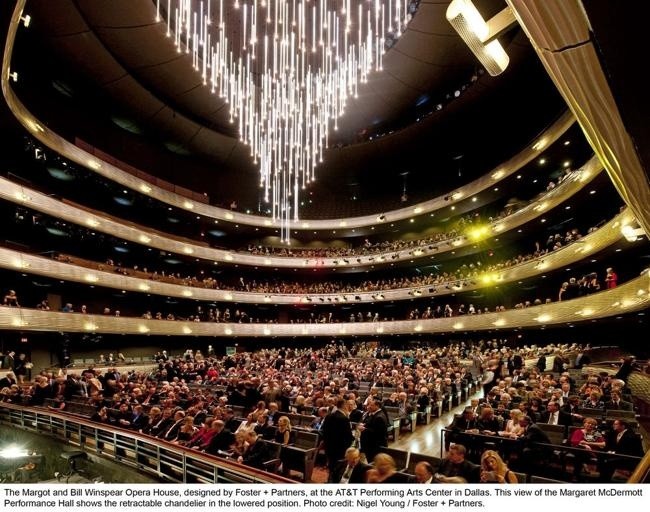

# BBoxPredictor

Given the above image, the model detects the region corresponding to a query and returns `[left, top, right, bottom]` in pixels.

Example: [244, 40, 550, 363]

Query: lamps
[9, 71, 18, 82]
[19, 15, 31, 28]
[150, 0, 414, 246]
[444, 0, 515, 79]
[622, 223, 645, 243]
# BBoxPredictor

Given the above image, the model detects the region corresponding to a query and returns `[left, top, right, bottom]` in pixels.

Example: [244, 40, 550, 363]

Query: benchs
[45, 381, 650, 483]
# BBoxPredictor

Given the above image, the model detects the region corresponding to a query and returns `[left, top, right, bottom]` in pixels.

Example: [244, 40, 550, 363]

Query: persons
[1, 166, 645, 482]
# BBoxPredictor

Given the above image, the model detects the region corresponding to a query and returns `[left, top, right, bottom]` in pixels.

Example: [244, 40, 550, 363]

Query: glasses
[622, 223, 645, 243]
[150, 0, 414, 246]
[444, 0, 515, 79]
[19, 15, 31, 28]
[9, 71, 18, 82]
